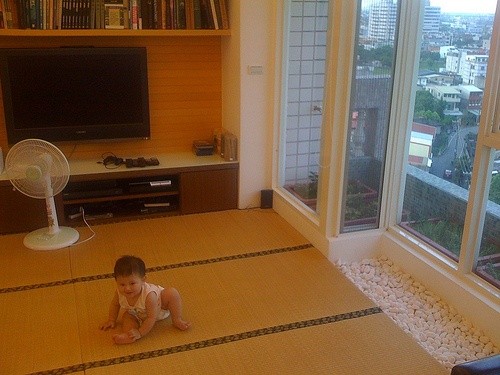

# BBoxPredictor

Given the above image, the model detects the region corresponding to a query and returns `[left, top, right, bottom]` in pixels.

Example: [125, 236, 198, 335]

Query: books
[141, 0, 202, 29]
[91, 0, 142, 30]
[200, 0, 229, 30]
[0, 0, 90, 29]
[213, 128, 237, 160]
[192, 139, 214, 156]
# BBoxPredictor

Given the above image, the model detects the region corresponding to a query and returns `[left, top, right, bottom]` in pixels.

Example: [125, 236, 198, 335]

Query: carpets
[0, 208, 452, 375]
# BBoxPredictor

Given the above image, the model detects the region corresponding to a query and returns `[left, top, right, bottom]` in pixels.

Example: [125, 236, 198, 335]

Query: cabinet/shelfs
[0, 152, 239, 235]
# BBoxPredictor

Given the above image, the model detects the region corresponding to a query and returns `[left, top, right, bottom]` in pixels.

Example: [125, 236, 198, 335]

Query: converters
[125, 160, 147, 168]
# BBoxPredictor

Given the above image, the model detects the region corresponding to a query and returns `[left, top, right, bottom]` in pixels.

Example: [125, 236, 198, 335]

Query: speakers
[260, 188, 274, 209]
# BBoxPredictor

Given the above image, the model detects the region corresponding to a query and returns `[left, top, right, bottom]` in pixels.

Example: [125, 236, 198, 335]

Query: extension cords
[68, 210, 85, 219]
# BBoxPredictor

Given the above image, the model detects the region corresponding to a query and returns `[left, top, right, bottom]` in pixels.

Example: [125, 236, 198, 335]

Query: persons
[98, 255, 190, 345]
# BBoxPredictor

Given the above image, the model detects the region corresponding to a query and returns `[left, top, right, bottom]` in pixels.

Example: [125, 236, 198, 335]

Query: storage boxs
[192, 140, 215, 156]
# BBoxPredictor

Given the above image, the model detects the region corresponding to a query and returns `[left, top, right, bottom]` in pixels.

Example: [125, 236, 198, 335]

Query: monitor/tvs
[0, 45, 152, 148]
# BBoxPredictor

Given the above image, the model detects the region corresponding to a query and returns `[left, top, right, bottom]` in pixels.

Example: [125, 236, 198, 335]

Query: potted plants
[283, 171, 500, 289]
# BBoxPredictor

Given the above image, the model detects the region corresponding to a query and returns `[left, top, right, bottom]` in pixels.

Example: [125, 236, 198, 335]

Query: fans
[5, 139, 80, 251]
